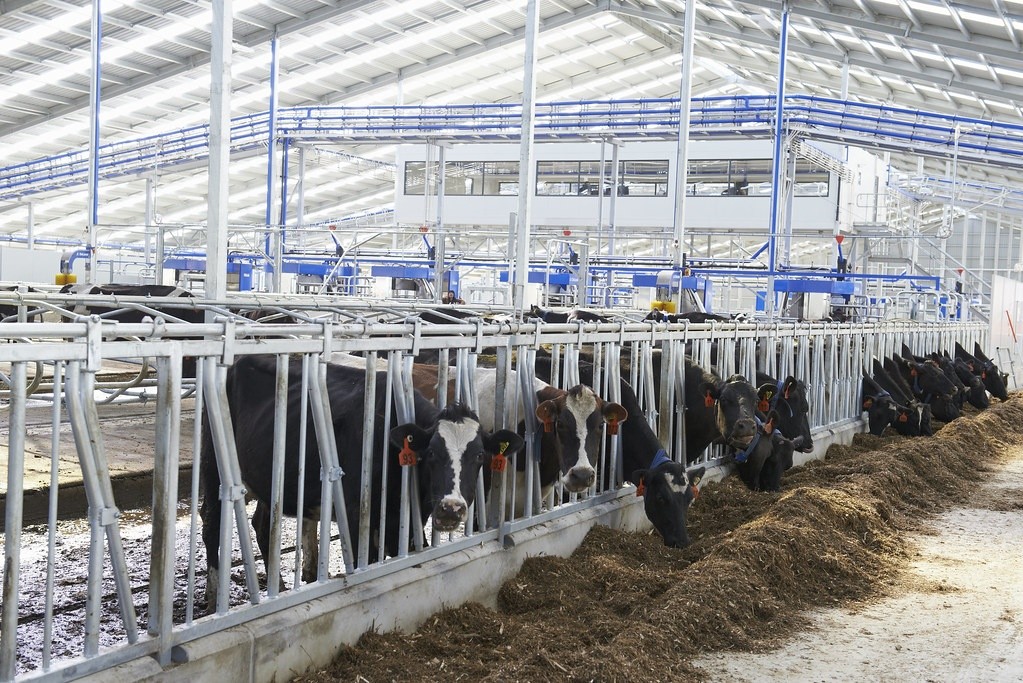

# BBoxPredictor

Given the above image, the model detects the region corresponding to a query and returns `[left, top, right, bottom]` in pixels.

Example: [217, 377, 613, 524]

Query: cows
[0, 282, 1011, 616]
